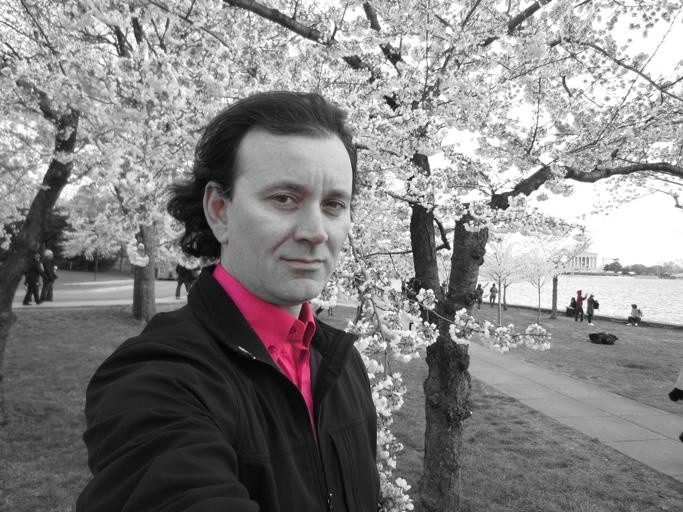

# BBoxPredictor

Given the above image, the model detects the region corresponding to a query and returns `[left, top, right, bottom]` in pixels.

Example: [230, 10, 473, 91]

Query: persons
[22, 251, 49, 305]
[490, 283, 498, 307]
[189, 264, 202, 281]
[475, 283, 483, 310]
[626, 303, 642, 326]
[574, 290, 587, 322]
[175, 264, 193, 299]
[569, 297, 576, 310]
[667, 367, 683, 444]
[75, 89, 381, 512]
[40, 248, 58, 301]
[587, 292, 595, 326]
